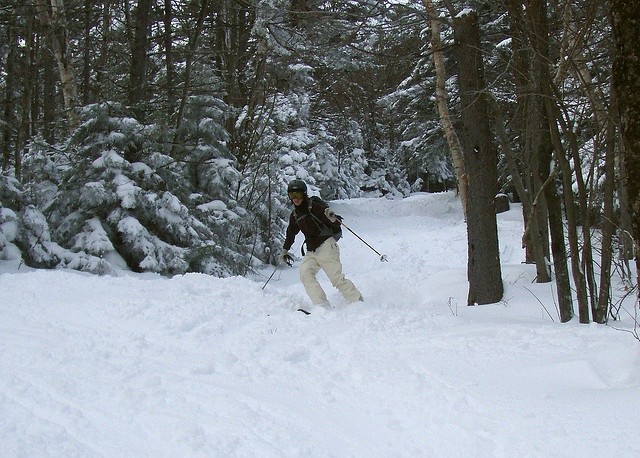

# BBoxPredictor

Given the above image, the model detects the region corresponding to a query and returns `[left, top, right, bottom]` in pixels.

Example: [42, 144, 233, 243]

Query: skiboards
[297, 309, 311, 315]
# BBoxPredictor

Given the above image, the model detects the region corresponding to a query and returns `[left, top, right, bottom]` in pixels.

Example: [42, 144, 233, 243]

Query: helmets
[288, 181, 307, 197]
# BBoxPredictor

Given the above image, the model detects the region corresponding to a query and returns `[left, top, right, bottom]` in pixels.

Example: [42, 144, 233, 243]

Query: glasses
[288, 191, 304, 200]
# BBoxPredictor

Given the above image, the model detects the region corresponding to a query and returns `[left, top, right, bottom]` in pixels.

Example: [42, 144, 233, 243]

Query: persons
[280, 180, 364, 309]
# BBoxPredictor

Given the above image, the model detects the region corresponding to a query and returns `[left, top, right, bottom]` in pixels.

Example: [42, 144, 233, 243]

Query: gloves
[277, 249, 290, 265]
[324, 208, 336, 223]
